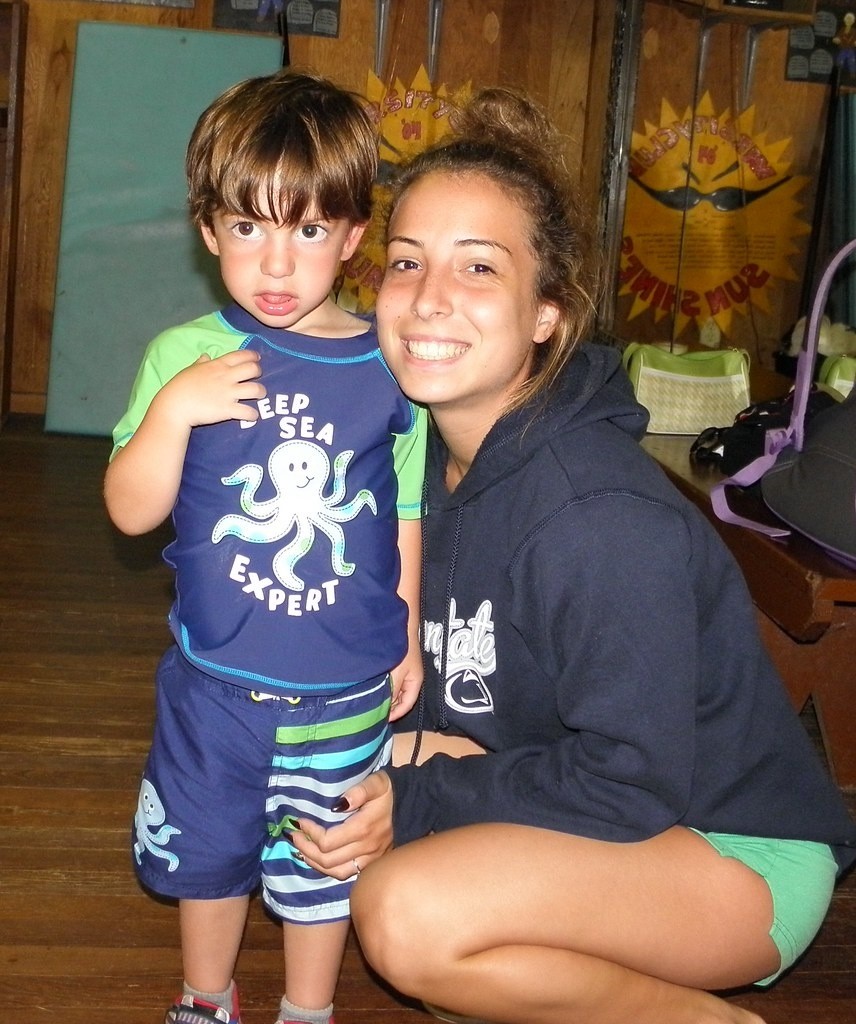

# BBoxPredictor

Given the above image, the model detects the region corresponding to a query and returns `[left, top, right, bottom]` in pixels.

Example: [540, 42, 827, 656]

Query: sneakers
[163, 984, 241, 1024]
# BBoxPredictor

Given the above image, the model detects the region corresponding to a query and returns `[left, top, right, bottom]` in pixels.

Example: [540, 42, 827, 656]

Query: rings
[351, 858, 361, 875]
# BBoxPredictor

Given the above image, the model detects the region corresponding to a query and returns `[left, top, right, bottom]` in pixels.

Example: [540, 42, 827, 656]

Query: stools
[637, 428, 856, 796]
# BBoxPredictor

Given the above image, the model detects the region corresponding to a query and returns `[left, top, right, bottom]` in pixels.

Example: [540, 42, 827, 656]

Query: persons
[103, 71, 429, 1024]
[282, 86, 855, 1024]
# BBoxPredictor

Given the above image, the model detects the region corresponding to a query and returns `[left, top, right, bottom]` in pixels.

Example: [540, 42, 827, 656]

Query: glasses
[690, 427, 733, 453]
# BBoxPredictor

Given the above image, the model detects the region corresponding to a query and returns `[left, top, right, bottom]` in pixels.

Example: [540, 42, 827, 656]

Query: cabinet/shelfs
[0, 0, 848, 419]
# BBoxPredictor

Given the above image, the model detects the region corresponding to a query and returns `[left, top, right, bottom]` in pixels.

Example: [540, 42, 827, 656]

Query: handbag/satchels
[819, 353, 856, 398]
[622, 340, 751, 434]
